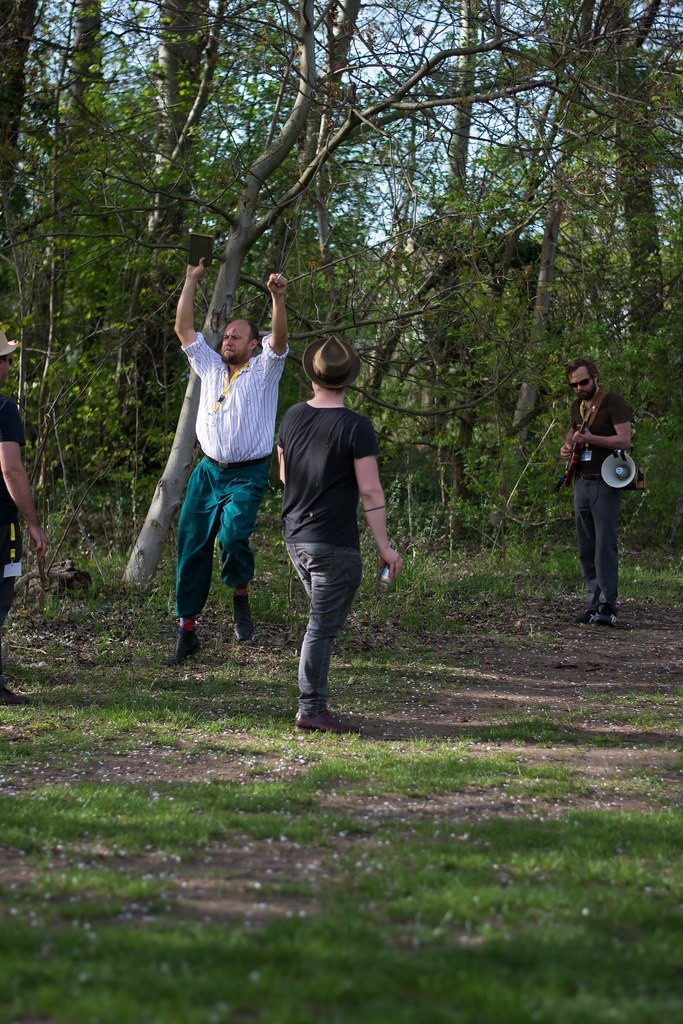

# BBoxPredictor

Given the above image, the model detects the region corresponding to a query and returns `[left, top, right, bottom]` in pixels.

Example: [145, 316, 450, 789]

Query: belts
[575, 473, 602, 480]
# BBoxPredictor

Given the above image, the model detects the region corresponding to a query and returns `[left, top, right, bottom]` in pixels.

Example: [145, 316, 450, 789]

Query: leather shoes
[295, 709, 361, 737]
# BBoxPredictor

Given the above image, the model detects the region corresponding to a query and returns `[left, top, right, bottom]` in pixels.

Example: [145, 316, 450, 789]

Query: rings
[36, 542, 42, 547]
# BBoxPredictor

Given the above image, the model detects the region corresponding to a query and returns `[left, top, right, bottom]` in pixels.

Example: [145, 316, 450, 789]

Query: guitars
[564, 406, 596, 488]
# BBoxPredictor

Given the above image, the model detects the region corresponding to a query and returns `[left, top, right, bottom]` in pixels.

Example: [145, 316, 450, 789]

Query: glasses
[568, 376, 593, 388]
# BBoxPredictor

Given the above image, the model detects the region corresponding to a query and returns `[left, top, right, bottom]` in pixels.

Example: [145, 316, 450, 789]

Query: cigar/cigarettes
[276, 272, 284, 279]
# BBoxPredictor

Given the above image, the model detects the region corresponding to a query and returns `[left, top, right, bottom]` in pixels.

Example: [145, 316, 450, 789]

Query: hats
[303, 336, 360, 389]
[0, 330, 18, 356]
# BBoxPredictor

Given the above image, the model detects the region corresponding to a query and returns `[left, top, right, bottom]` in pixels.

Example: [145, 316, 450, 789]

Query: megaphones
[601, 452, 639, 489]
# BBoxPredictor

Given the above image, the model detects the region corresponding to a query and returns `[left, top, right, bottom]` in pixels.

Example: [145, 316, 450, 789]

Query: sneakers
[166, 633, 199, 665]
[233, 593, 253, 641]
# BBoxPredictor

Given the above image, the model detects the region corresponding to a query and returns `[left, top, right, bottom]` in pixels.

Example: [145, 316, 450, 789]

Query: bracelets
[365, 505, 385, 512]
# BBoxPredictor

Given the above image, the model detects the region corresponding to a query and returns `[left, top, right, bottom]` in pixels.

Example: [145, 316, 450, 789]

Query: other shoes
[574, 610, 600, 624]
[594, 614, 615, 624]
[0, 685, 29, 704]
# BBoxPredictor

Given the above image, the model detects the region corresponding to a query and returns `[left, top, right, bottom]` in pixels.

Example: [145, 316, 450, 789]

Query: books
[188, 232, 212, 266]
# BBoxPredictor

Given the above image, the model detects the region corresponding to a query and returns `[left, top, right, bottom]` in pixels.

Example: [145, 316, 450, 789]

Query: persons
[275, 337, 404, 736]
[559, 357, 635, 628]
[0, 326, 48, 706]
[174, 256, 289, 661]
